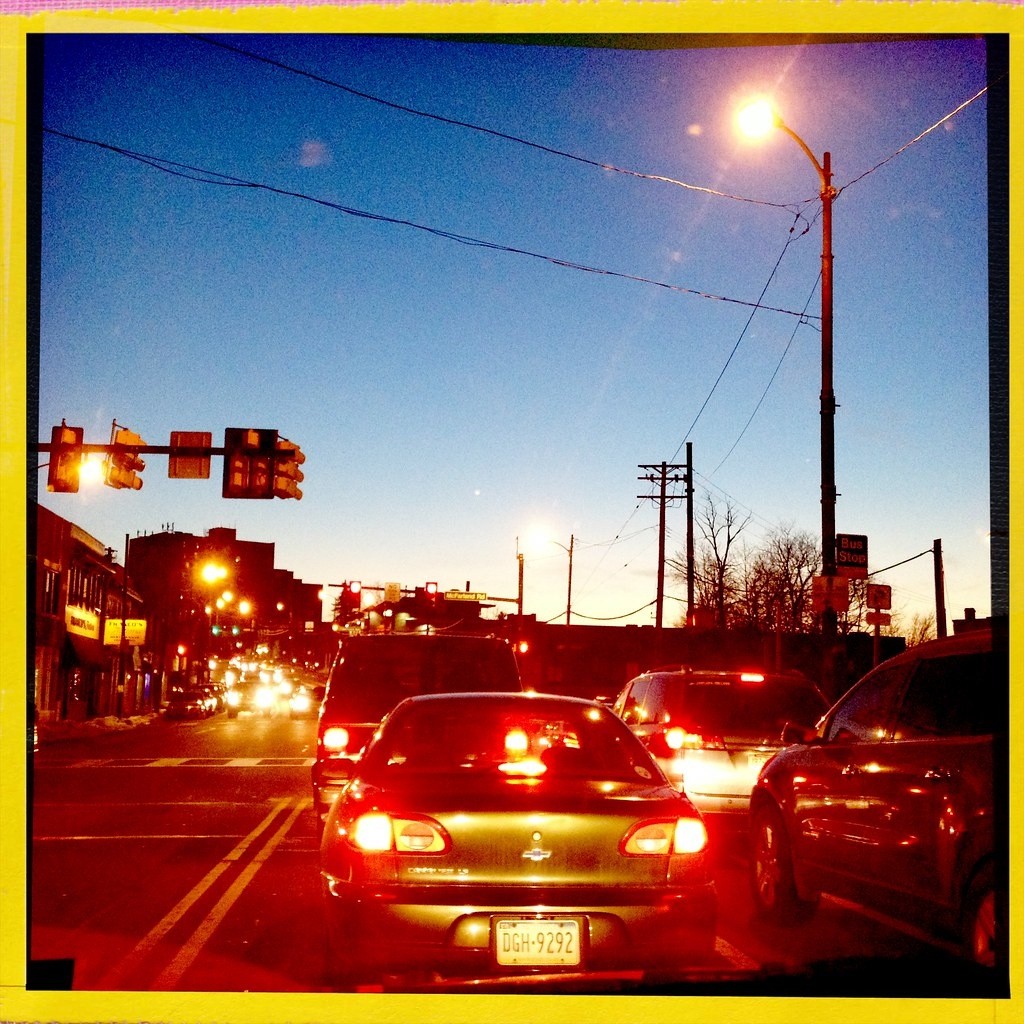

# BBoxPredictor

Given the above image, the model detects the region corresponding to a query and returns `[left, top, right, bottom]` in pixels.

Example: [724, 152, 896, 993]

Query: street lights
[728, 92, 837, 706]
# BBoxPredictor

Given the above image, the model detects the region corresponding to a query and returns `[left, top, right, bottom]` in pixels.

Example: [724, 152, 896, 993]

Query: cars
[167, 646, 624, 722]
[317, 693, 714, 993]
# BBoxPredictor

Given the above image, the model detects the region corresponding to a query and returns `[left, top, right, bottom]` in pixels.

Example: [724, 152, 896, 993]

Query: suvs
[747, 624, 1002, 984]
[311, 631, 539, 844]
[605, 664, 840, 820]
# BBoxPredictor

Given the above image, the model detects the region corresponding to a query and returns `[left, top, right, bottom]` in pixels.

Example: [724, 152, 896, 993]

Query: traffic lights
[111, 429, 148, 490]
[274, 438, 306, 501]
